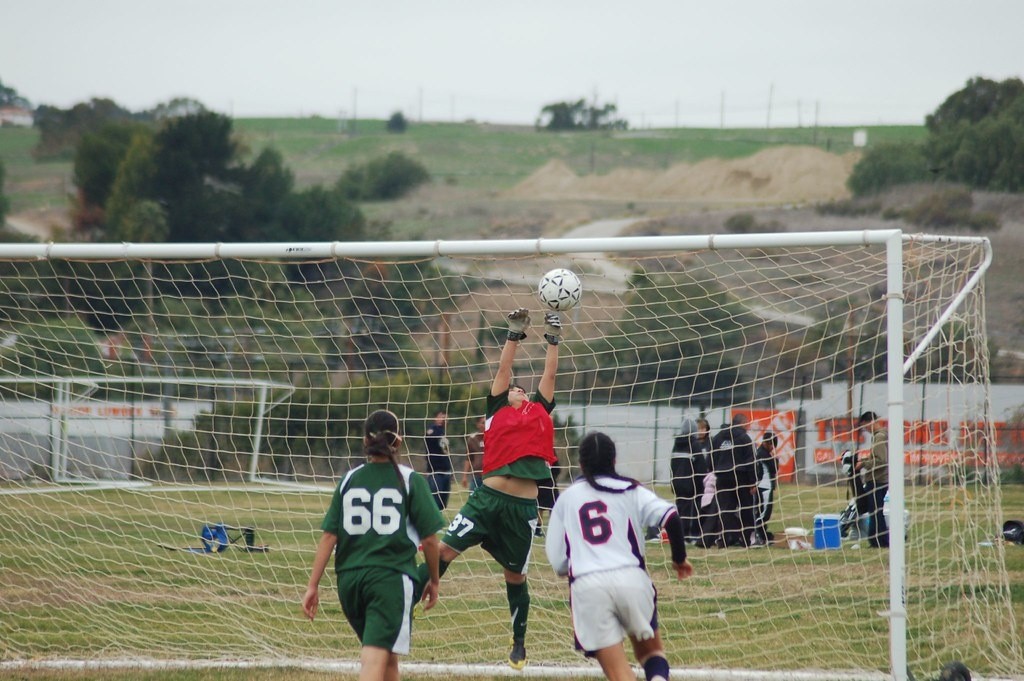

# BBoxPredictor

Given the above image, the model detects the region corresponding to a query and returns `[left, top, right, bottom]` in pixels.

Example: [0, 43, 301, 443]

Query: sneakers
[507, 636, 526, 669]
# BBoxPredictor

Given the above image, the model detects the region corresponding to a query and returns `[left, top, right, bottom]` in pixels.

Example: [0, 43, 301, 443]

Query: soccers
[539, 267, 583, 311]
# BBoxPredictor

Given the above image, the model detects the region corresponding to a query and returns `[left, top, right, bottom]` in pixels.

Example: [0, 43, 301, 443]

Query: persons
[546, 430, 694, 681]
[302, 409, 447, 681]
[422, 408, 561, 540]
[840, 412, 889, 550]
[670, 413, 779, 549]
[415, 307, 561, 665]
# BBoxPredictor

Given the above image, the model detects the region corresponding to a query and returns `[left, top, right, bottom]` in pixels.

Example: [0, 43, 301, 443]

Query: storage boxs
[812, 513, 842, 549]
[772, 530, 813, 548]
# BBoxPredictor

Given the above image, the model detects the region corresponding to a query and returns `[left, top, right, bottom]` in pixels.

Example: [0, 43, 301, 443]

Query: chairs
[183, 528, 248, 555]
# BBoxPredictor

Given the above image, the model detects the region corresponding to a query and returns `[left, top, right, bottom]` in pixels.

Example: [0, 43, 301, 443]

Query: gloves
[543, 312, 562, 346]
[506, 310, 533, 342]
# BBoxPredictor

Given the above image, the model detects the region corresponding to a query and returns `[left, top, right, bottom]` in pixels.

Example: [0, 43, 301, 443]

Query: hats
[682, 417, 698, 436]
